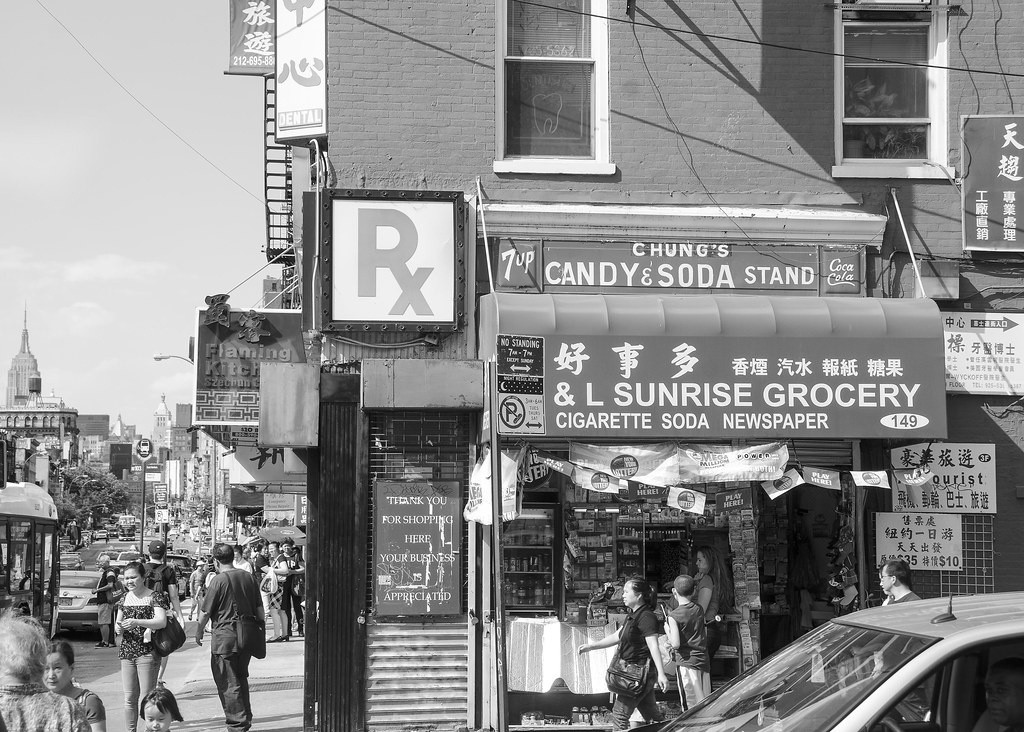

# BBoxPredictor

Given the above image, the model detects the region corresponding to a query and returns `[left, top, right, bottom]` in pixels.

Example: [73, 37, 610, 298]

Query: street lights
[82, 480, 100, 490]
[152, 354, 217, 551]
[68, 474, 89, 498]
[20, 451, 48, 483]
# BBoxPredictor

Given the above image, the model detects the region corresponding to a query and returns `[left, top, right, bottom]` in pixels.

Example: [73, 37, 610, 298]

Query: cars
[42, 570, 103, 636]
[63, 514, 237, 602]
[59, 551, 86, 571]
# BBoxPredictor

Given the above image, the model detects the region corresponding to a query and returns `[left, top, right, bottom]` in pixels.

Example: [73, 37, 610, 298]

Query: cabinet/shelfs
[502, 544, 554, 612]
[655, 614, 739, 691]
[568, 511, 727, 593]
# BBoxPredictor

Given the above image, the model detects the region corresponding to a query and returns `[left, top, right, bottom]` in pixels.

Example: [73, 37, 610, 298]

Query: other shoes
[299, 631, 304, 637]
[94, 642, 109, 649]
[188, 614, 192, 620]
[288, 630, 292, 636]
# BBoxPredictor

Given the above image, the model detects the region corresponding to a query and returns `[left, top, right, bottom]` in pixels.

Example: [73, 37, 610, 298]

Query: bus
[0, 482, 63, 644]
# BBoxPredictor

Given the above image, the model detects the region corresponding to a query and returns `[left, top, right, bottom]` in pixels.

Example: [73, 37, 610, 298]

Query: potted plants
[845, 74, 927, 158]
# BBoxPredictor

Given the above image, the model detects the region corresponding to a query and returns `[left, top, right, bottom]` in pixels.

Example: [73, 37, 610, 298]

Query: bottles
[503, 524, 553, 606]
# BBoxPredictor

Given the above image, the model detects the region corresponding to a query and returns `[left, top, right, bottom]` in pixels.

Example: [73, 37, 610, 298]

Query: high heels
[282, 635, 289, 641]
[266, 636, 282, 643]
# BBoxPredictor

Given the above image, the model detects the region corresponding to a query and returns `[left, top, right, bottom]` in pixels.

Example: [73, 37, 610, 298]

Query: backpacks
[142, 563, 168, 591]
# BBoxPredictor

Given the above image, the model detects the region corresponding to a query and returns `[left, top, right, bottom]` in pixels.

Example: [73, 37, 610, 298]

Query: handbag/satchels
[605, 650, 651, 700]
[236, 614, 266, 659]
[150, 591, 186, 657]
[292, 574, 305, 596]
[105, 569, 125, 605]
[259, 569, 278, 595]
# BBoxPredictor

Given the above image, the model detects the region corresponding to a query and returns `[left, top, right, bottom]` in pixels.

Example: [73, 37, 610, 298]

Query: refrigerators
[491, 501, 562, 619]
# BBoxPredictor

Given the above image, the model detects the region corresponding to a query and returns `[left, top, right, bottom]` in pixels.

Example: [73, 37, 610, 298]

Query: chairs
[972, 640, 1024, 732]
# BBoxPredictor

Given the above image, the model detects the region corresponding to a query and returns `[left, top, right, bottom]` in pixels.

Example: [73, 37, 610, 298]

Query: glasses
[881, 575, 894, 579]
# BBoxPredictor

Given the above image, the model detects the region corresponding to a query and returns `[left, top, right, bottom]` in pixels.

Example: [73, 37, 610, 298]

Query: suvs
[657, 590, 1024, 732]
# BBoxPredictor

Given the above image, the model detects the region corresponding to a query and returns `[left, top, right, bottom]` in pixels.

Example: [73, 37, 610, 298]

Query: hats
[280, 537, 294, 547]
[196, 560, 206, 566]
[826, 525, 859, 615]
[149, 540, 166, 555]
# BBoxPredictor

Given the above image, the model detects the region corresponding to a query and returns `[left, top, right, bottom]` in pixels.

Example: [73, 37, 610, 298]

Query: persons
[189, 537, 306, 646]
[664, 574, 711, 710]
[196, 543, 265, 732]
[140, 681, 183, 732]
[41, 641, 106, 732]
[578, 577, 670, 732]
[851, 558, 938, 723]
[986, 656, 1024, 732]
[105, 532, 110, 544]
[171, 515, 211, 545]
[141, 540, 185, 689]
[115, 562, 167, 732]
[0, 608, 92, 732]
[663, 545, 732, 694]
[92, 554, 115, 649]
